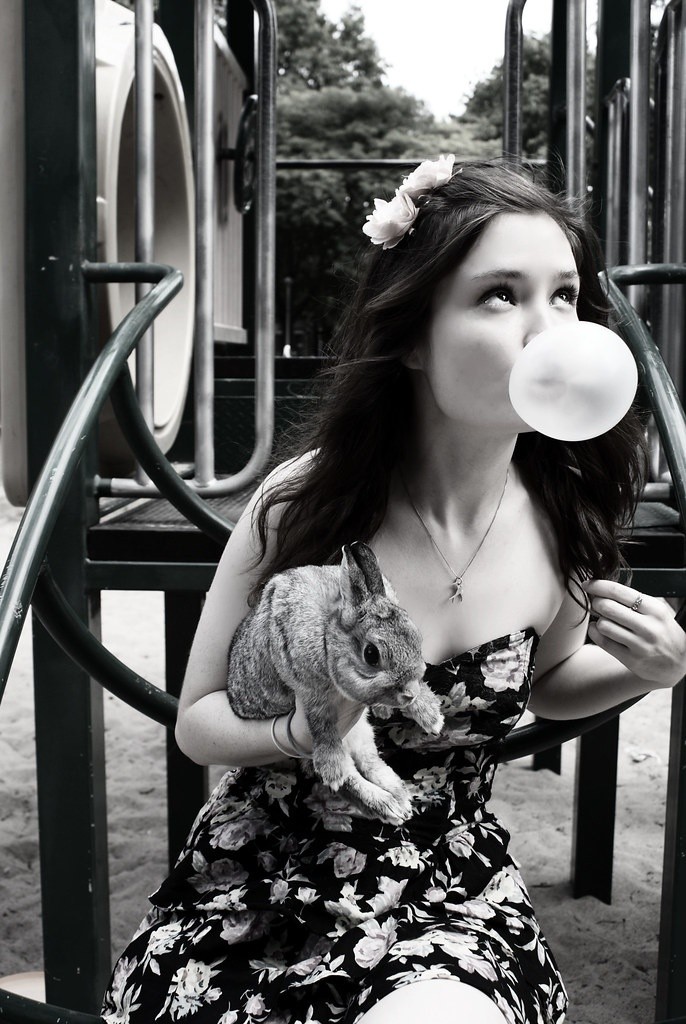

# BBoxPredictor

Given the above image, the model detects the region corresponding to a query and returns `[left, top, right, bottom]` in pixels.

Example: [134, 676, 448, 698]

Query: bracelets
[285, 708, 313, 759]
[270, 716, 304, 758]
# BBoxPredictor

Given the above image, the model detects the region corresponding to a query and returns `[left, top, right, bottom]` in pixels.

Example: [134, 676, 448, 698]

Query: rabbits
[226, 539, 445, 826]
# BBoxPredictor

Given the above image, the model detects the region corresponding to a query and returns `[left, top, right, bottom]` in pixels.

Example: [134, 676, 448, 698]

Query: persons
[99, 164, 686, 1024]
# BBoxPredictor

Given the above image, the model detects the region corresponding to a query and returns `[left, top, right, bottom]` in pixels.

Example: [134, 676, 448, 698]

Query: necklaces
[403, 467, 509, 603]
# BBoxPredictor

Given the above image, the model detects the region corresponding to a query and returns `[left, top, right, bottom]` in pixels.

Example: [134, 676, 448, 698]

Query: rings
[632, 593, 645, 610]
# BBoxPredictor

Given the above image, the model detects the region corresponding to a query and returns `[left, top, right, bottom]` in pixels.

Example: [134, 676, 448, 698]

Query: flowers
[362, 192, 421, 251]
[398, 154, 462, 198]
